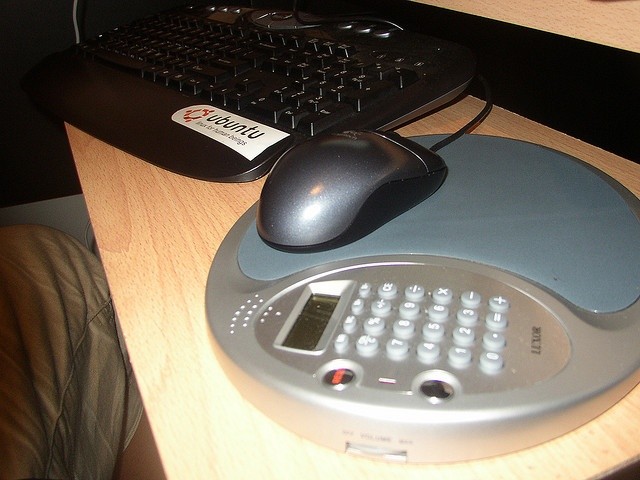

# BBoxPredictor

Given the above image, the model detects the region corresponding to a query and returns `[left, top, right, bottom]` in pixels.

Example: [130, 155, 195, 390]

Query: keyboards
[25, 11, 474, 183]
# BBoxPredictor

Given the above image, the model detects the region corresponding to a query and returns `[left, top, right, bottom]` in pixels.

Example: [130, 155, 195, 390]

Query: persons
[2, 222, 168, 480]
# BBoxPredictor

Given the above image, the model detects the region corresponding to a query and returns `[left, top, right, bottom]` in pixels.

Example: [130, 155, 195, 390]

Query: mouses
[256, 130, 446, 254]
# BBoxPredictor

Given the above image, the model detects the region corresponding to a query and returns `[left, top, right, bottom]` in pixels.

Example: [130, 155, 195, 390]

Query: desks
[64, 92, 640, 480]
